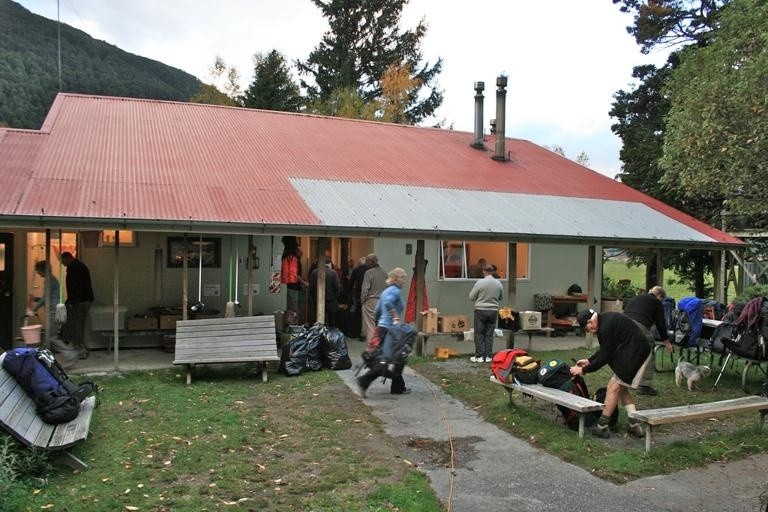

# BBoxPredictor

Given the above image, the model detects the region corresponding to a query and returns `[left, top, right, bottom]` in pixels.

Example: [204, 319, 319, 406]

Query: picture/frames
[167, 236, 221, 268]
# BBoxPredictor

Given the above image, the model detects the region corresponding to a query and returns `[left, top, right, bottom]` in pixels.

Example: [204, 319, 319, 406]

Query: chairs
[655, 297, 768, 392]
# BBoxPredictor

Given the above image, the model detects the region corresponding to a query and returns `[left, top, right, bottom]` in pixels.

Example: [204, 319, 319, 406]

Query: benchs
[0, 359, 97, 471]
[490, 376, 607, 439]
[631, 395, 768, 454]
[418, 329, 520, 357]
[103, 330, 175, 353]
[172, 315, 280, 385]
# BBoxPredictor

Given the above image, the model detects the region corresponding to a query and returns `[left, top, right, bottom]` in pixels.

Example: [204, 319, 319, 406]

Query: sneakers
[391, 387, 412, 395]
[589, 424, 609, 439]
[485, 356, 492, 363]
[624, 422, 645, 439]
[636, 385, 657, 396]
[354, 378, 368, 399]
[470, 356, 485, 363]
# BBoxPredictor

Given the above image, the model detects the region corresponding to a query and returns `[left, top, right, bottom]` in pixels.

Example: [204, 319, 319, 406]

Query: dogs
[674, 356, 714, 391]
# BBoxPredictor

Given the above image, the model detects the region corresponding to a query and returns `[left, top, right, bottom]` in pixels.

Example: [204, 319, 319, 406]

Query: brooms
[223, 234, 236, 319]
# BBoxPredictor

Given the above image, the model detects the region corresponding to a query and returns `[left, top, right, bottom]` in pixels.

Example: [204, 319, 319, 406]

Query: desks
[535, 295, 596, 337]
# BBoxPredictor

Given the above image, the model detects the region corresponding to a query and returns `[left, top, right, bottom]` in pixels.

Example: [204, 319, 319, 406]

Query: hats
[576, 309, 595, 328]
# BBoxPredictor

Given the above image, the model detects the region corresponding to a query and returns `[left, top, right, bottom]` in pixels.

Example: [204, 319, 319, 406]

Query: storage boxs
[419, 307, 440, 333]
[89, 306, 190, 331]
[519, 311, 542, 328]
[438, 314, 468, 332]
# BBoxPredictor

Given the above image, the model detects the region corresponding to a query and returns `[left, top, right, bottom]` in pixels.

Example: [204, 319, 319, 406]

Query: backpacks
[594, 388, 620, 433]
[352, 322, 418, 384]
[536, 357, 594, 434]
[646, 295, 768, 366]
[1, 345, 96, 425]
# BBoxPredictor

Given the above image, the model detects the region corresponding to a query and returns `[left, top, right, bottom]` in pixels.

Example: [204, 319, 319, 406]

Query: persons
[62, 252, 94, 359]
[468, 265, 504, 363]
[353, 267, 411, 398]
[287, 247, 389, 352]
[569, 309, 653, 438]
[457, 259, 501, 279]
[33, 261, 60, 340]
[623, 286, 673, 396]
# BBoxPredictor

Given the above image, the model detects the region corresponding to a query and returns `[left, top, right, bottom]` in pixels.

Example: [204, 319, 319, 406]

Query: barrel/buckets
[18, 312, 44, 345]
